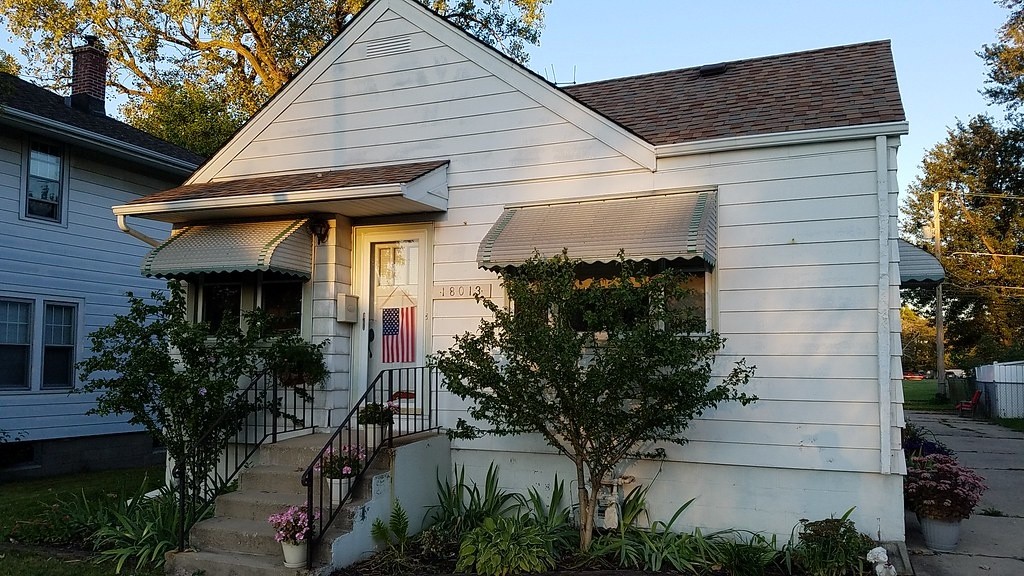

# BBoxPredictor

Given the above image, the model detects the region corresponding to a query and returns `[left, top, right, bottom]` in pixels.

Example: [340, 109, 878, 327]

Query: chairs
[956, 390, 982, 420]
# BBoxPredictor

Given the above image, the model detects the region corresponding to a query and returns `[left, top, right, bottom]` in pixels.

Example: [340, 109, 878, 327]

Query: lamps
[307, 217, 334, 246]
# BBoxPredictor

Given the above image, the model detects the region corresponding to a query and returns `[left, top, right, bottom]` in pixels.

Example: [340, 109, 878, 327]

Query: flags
[382, 305, 416, 363]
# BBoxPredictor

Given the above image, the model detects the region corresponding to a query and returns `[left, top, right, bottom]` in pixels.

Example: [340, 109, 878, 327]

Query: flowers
[903, 452, 991, 523]
[292, 443, 369, 479]
[359, 401, 399, 426]
[386, 390, 415, 402]
[269, 502, 325, 548]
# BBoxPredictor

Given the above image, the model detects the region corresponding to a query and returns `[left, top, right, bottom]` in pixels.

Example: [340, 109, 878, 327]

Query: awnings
[476, 185, 717, 272]
[900, 237, 946, 289]
[141, 219, 313, 280]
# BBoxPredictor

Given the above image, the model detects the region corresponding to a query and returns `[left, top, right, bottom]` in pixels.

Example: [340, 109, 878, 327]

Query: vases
[920, 515, 962, 551]
[393, 398, 414, 414]
[326, 475, 357, 505]
[281, 538, 308, 569]
[363, 423, 387, 448]
